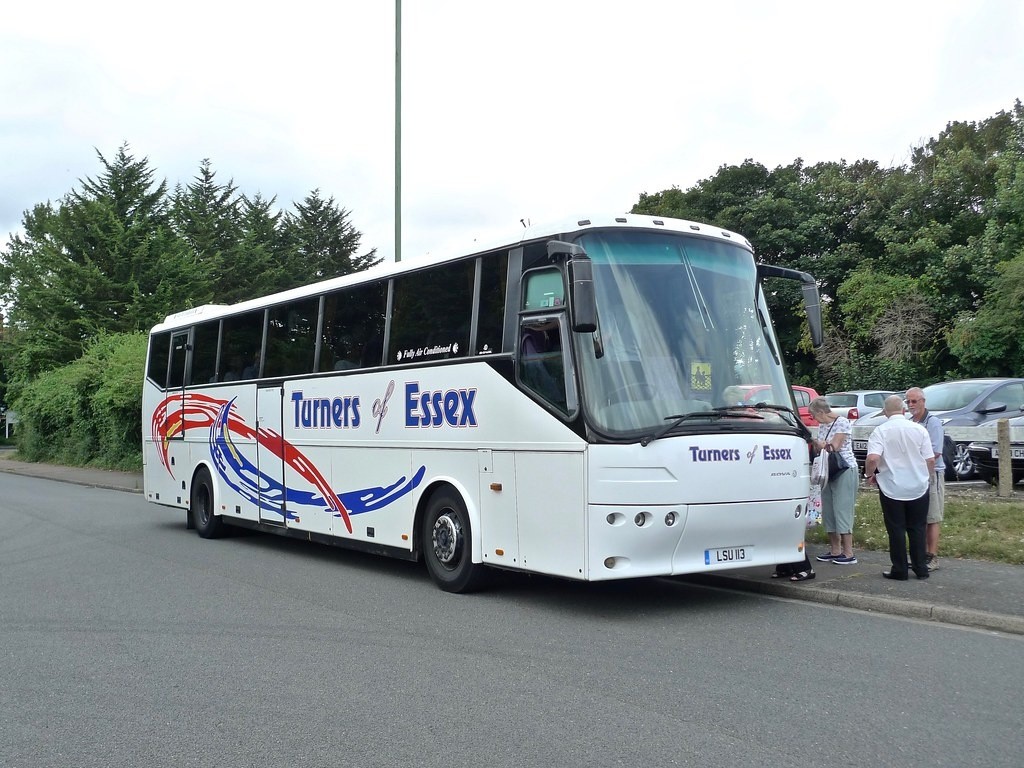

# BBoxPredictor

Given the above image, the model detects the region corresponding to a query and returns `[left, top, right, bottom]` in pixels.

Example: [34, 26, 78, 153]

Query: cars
[965, 407, 1024, 481]
[726, 385, 823, 460]
[826, 389, 908, 429]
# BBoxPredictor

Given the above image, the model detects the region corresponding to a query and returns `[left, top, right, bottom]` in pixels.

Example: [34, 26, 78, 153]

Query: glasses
[903, 398, 924, 404]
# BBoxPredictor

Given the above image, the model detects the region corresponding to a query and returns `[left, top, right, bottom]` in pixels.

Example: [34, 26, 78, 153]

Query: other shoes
[883, 571, 897, 579]
[916, 574, 929, 580]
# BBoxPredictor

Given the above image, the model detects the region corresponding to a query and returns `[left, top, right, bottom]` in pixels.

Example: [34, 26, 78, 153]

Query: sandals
[771, 570, 792, 578]
[790, 569, 815, 581]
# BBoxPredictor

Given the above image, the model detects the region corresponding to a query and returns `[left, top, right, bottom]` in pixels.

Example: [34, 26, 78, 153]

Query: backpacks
[926, 416, 957, 478]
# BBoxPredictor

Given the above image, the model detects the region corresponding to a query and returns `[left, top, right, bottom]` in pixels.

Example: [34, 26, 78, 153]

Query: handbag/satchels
[811, 442, 849, 491]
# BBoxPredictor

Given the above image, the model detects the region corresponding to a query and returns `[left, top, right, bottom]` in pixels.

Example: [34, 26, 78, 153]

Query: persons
[771, 550, 816, 581]
[904, 387, 946, 572]
[334, 337, 382, 370]
[864, 395, 936, 581]
[807, 396, 857, 564]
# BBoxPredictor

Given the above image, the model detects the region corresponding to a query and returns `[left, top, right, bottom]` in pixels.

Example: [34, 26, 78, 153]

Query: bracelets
[864, 473, 871, 479]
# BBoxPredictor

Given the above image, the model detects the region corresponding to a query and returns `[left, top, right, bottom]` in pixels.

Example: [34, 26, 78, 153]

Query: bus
[141, 214, 825, 596]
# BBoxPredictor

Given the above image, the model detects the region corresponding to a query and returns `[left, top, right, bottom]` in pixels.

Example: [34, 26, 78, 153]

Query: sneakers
[908, 552, 940, 572]
[833, 555, 857, 564]
[817, 552, 843, 561]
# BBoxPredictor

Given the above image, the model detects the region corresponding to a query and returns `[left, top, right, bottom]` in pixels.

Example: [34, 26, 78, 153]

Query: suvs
[849, 378, 1023, 481]
